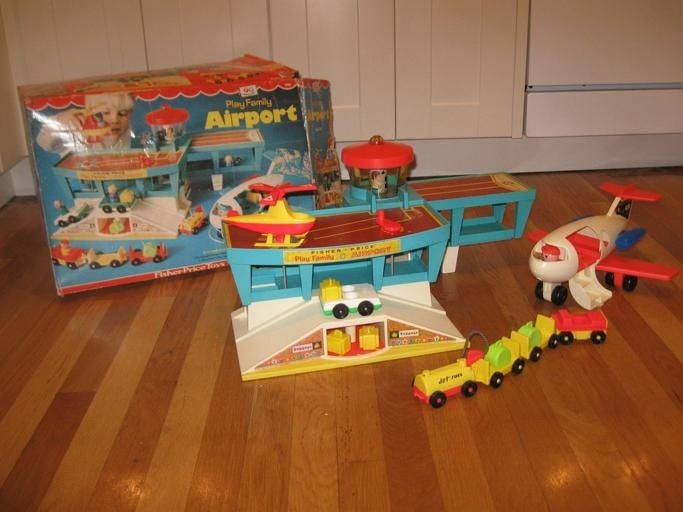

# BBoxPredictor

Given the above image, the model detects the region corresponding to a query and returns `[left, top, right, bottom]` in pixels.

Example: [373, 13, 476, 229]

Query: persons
[35, 82, 136, 158]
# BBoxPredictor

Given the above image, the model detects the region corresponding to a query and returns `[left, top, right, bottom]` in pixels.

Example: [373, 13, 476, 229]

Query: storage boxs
[14, 53, 347, 305]
